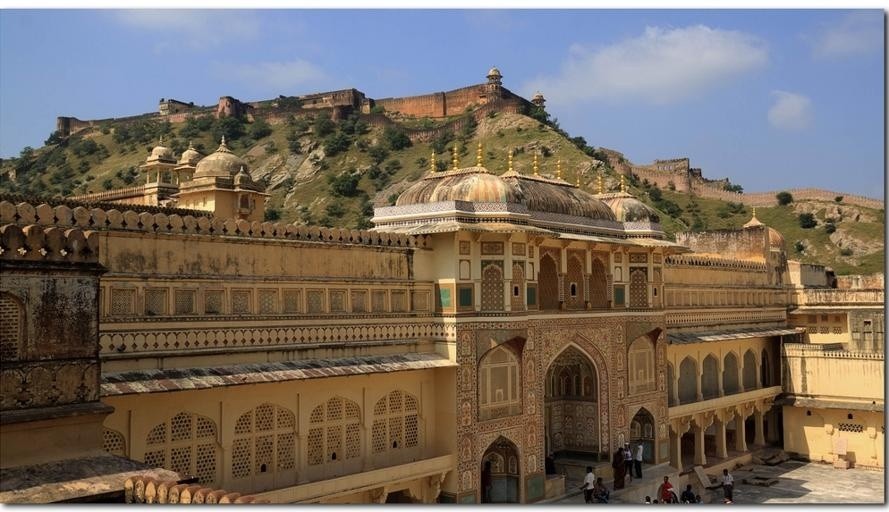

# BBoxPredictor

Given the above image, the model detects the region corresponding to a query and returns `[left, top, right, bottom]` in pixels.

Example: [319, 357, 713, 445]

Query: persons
[612, 447, 625, 489]
[622, 441, 634, 482]
[480, 460, 493, 502]
[578, 465, 596, 503]
[592, 476, 610, 503]
[543, 453, 559, 475]
[720, 468, 734, 503]
[659, 475, 674, 500]
[645, 483, 704, 504]
[633, 438, 644, 479]
